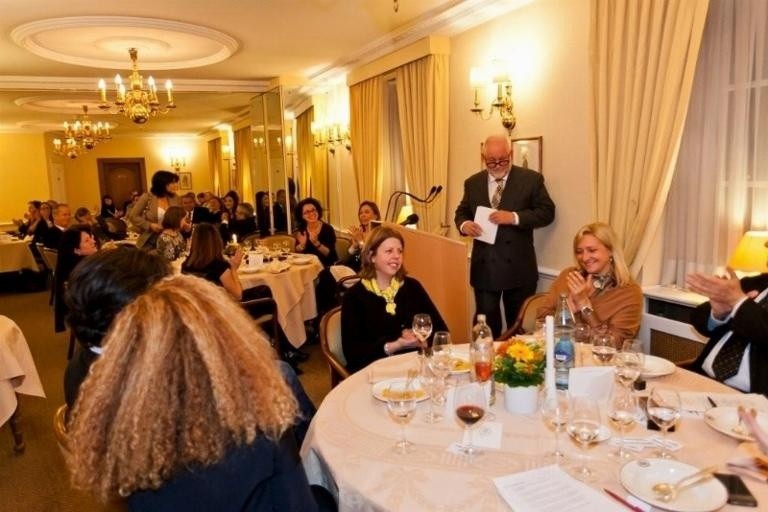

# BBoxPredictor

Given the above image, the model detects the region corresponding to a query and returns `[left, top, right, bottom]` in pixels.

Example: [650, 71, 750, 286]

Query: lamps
[49, 50, 175, 160]
[470, 70, 517, 130]
[310, 122, 341, 157]
[717, 232, 768, 278]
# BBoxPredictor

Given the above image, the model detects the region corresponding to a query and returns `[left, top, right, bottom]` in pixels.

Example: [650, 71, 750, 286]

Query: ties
[711, 287, 767, 382]
[491, 177, 504, 208]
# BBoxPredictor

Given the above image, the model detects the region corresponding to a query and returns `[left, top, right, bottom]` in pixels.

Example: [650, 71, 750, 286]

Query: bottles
[467, 314, 494, 383]
[552, 333, 574, 389]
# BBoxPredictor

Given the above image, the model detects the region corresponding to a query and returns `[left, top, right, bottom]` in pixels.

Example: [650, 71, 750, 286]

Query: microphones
[399, 213, 419, 226]
[384, 185, 436, 221]
[390, 185, 443, 223]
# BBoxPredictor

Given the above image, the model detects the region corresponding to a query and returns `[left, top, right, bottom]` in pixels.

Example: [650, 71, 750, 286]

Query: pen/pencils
[602, 488, 645, 512]
[707, 396, 717, 408]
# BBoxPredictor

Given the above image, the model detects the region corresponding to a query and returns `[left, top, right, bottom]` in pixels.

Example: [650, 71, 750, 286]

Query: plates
[623, 351, 675, 378]
[621, 454, 727, 512]
[427, 350, 471, 375]
[372, 377, 428, 403]
[704, 405, 767, 444]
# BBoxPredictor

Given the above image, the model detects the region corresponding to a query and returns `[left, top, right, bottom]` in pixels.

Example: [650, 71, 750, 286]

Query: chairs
[256, 235, 297, 253]
[36, 243, 59, 307]
[333, 237, 352, 265]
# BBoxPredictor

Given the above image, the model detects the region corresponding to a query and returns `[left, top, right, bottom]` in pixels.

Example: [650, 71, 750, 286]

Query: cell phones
[713, 473, 757, 507]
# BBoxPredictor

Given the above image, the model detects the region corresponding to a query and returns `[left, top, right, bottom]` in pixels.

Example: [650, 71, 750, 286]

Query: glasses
[483, 155, 510, 168]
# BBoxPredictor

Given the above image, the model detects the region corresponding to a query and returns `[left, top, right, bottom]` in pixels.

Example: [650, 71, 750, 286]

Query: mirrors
[249, 84, 330, 238]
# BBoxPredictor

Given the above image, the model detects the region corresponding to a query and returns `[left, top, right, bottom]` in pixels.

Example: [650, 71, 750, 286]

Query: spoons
[650, 462, 716, 496]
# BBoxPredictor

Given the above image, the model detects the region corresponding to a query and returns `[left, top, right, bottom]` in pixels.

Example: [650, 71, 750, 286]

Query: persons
[454, 132, 556, 341]
[63, 244, 318, 453]
[534, 221, 646, 352]
[684, 237, 767, 400]
[338, 224, 453, 372]
[7, 167, 383, 378]
[58, 272, 341, 511]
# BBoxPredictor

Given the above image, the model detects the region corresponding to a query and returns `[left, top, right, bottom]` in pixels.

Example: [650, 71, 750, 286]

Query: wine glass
[452, 377, 487, 458]
[386, 380, 419, 455]
[411, 314, 452, 424]
[542, 321, 682, 484]
[222, 234, 315, 274]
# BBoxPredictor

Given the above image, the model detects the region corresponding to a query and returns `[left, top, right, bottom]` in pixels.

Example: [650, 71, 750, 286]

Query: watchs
[579, 305, 594, 316]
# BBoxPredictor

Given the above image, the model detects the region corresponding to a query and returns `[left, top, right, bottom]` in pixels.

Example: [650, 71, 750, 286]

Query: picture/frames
[179, 171, 192, 190]
[513, 135, 543, 177]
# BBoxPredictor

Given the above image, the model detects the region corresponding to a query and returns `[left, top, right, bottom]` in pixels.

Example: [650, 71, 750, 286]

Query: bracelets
[383, 343, 391, 356]
